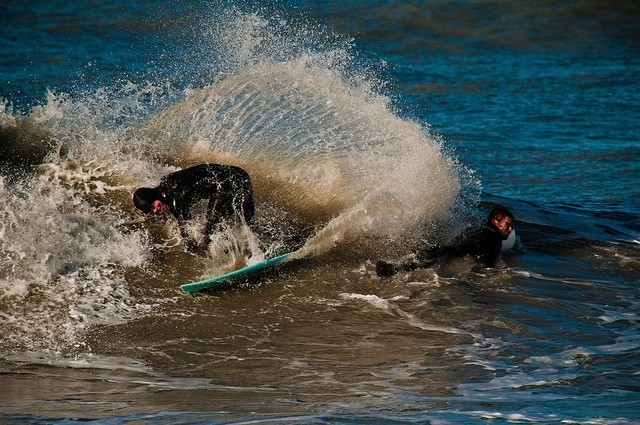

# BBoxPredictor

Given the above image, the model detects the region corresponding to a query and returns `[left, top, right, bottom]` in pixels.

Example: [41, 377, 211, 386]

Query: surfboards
[180, 252, 293, 292]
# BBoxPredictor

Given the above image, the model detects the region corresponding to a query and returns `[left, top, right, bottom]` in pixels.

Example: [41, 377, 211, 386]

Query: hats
[133, 187, 160, 214]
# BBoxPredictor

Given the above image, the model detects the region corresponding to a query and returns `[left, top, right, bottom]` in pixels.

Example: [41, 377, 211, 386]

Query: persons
[134, 164, 255, 250]
[376, 208, 515, 280]
